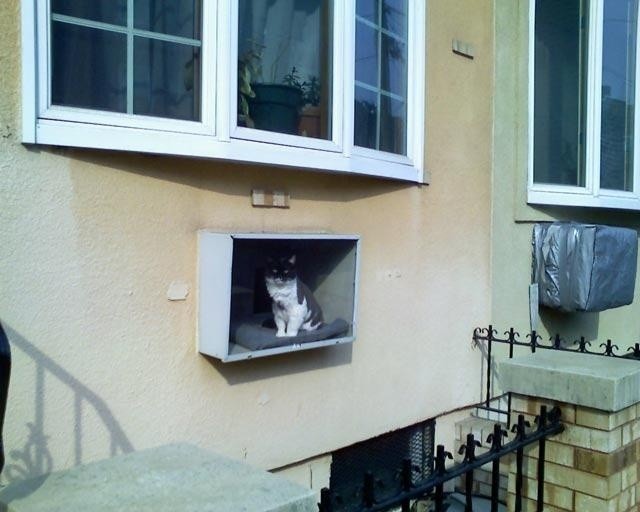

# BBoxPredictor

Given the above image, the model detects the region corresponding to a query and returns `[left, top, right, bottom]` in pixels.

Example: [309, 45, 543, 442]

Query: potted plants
[238, 30, 320, 138]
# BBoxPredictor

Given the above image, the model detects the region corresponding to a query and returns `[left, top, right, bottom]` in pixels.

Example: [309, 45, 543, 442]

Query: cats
[261, 253, 323, 338]
